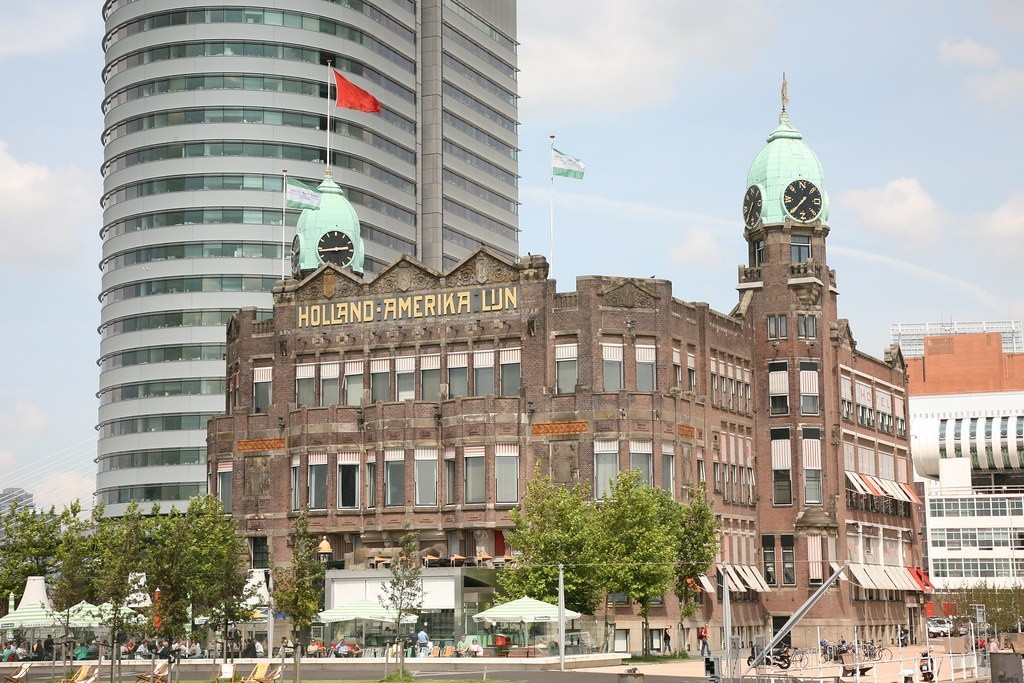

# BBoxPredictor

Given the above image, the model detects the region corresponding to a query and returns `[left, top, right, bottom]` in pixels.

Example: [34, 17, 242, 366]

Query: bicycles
[816, 638, 893, 664]
[780, 641, 808, 668]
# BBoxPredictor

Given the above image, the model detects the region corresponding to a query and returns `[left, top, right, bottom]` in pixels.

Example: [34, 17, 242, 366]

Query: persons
[407, 626, 433, 657]
[352, 638, 363, 657]
[701, 624, 712, 656]
[214, 626, 265, 657]
[663, 628, 672, 654]
[124, 637, 202, 659]
[0, 634, 112, 662]
[334, 639, 348, 658]
[457, 635, 469, 657]
[901, 630, 906, 646]
[277, 637, 304, 658]
[307, 639, 318, 657]
[463, 638, 483, 656]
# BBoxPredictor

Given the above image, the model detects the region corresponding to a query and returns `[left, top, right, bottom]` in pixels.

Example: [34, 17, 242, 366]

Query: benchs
[839, 653, 873, 677]
[473, 654, 492, 657]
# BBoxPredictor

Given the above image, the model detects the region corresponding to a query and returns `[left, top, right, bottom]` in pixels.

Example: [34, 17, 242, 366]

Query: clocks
[743, 186, 763, 227]
[318, 231, 354, 267]
[290, 235, 300, 272]
[783, 180, 823, 221]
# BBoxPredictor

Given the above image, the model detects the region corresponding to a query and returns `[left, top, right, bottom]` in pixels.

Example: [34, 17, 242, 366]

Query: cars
[926, 615, 989, 638]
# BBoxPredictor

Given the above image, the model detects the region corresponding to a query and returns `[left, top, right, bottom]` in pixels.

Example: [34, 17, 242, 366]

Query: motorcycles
[747, 645, 791, 670]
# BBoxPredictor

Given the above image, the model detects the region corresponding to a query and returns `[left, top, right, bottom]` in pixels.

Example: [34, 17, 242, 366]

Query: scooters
[918, 646, 935, 681]
[897, 629, 909, 648]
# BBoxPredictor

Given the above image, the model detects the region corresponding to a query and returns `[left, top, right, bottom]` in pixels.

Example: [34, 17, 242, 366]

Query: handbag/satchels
[697, 631, 703, 639]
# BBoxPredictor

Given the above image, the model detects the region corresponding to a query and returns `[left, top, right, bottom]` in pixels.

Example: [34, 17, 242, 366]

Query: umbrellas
[472, 594, 581, 646]
[311, 598, 419, 648]
[0, 599, 149, 653]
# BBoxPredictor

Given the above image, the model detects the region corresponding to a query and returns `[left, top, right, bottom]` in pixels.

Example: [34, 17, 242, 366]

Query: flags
[332, 68, 382, 113]
[285, 176, 321, 211]
[550, 144, 585, 180]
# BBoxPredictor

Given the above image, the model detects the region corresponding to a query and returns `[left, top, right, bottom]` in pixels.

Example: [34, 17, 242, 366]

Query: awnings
[830, 562, 848, 582]
[847, 562, 936, 591]
[695, 563, 772, 593]
[845, 470, 923, 506]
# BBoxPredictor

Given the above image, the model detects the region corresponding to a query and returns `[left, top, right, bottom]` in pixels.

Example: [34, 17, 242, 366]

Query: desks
[454, 649, 480, 657]
[501, 645, 537, 657]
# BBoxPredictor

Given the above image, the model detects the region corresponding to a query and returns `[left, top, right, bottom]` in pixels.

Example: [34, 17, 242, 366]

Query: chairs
[442, 646, 454, 657]
[61, 665, 102, 683]
[217, 662, 290, 683]
[1, 663, 32, 683]
[427, 646, 440, 657]
[6, 653, 16, 662]
[135, 662, 176, 683]
